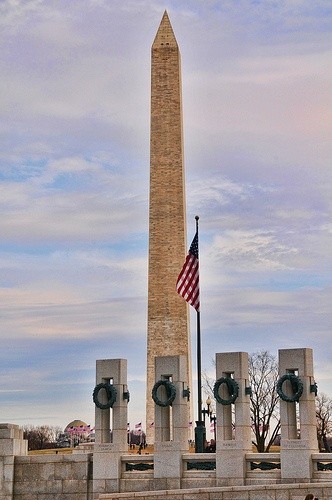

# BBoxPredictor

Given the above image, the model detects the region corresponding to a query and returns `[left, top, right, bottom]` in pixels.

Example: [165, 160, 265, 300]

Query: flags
[176, 230, 201, 313]
[64, 420, 262, 439]
